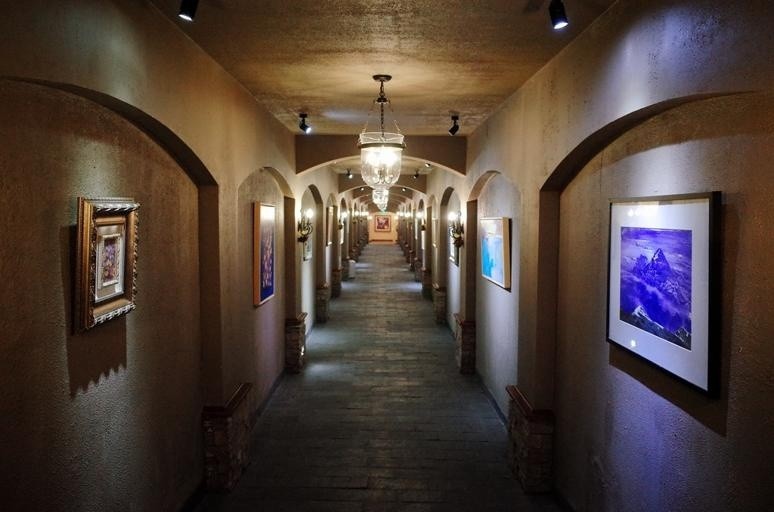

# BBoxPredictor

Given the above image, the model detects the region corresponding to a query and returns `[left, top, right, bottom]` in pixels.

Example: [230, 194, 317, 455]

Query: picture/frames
[327, 206, 334, 246]
[603, 189, 725, 407]
[76, 193, 142, 329]
[477, 215, 511, 290]
[252, 201, 276, 308]
[373, 213, 392, 232]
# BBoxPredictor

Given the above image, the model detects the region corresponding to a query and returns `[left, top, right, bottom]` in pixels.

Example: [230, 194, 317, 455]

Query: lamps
[296, 113, 312, 134]
[298, 207, 315, 244]
[446, 210, 464, 246]
[547, 0, 569, 30]
[177, 0, 197, 23]
[358, 74, 405, 211]
[446, 114, 459, 137]
